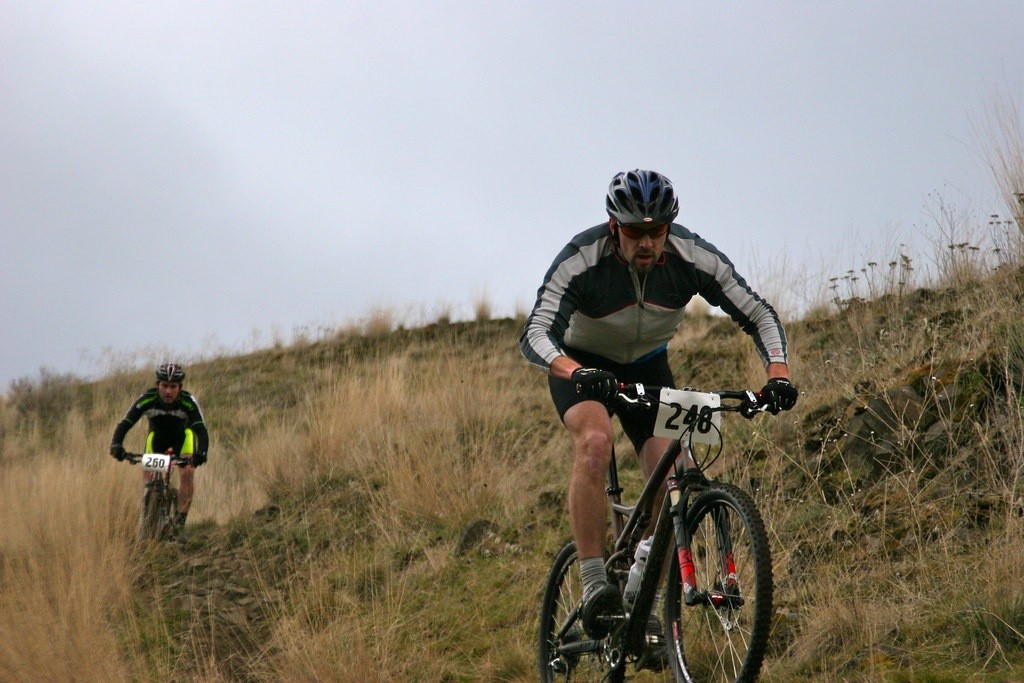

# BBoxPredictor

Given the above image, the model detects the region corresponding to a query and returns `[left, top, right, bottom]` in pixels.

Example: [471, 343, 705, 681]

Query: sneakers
[579, 582, 620, 640]
[640, 616, 668, 670]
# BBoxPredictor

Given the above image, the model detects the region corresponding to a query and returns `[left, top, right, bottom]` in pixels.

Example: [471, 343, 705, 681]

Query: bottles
[623, 535, 653, 608]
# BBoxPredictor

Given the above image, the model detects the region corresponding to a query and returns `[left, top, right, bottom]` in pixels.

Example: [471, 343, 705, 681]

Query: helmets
[156, 362, 185, 383]
[605, 168, 680, 229]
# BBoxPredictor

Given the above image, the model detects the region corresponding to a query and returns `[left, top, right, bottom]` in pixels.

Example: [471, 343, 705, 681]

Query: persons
[108, 362, 214, 536]
[517, 169, 799, 674]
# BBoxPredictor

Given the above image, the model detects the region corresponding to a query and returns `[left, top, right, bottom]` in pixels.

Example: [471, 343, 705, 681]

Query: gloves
[189, 451, 207, 468]
[110, 445, 127, 462]
[572, 368, 619, 404]
[760, 377, 798, 415]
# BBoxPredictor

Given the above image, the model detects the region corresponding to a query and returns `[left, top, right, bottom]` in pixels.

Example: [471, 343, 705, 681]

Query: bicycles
[116, 448, 207, 551]
[535, 379, 795, 683]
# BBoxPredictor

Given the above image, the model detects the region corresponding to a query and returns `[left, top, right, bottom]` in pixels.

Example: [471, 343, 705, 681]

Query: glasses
[616, 222, 668, 239]
